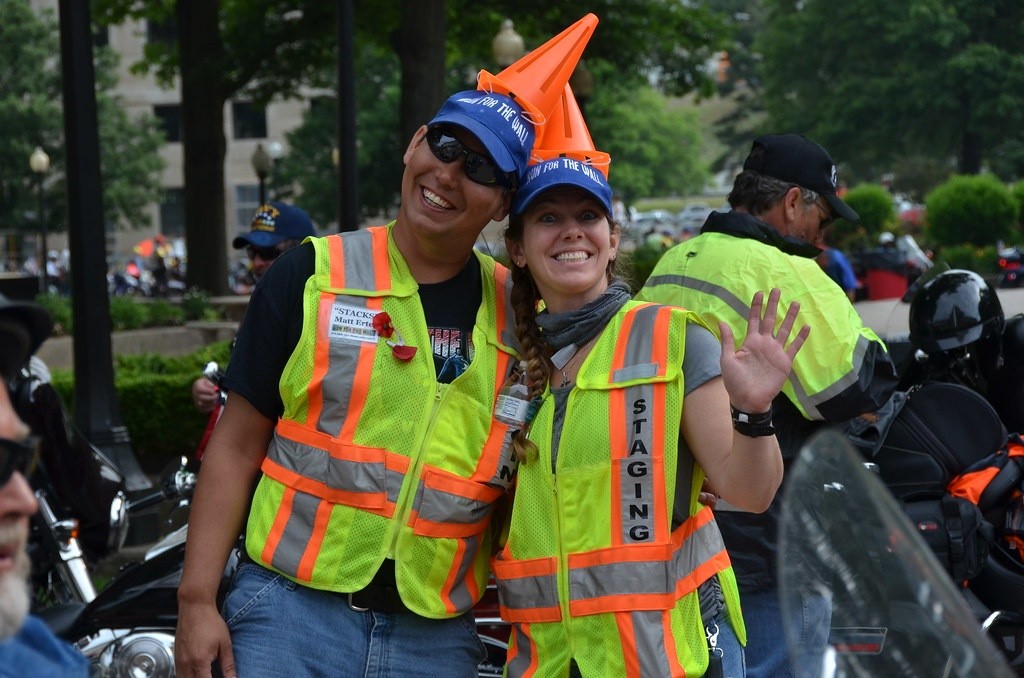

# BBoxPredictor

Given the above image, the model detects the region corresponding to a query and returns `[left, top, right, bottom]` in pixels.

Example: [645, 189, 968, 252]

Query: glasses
[1, 432, 42, 490]
[426, 125, 512, 190]
[243, 246, 284, 261]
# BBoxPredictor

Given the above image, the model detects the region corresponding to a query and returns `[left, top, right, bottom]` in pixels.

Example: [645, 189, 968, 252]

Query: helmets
[908, 269, 1006, 379]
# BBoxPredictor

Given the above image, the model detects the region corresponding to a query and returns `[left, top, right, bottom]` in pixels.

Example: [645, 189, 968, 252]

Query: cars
[632, 204, 731, 247]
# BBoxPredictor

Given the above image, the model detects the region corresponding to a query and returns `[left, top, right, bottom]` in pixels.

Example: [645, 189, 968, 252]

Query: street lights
[29, 146, 52, 292]
[252, 144, 271, 203]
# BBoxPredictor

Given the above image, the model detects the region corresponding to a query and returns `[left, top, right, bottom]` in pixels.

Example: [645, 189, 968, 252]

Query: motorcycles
[23, 358, 247, 678]
[26, 238, 253, 297]
[849, 234, 933, 292]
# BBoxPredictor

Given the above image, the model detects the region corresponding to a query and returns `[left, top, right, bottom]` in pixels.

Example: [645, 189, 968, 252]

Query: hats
[428, 90, 536, 182]
[511, 156, 614, 222]
[1, 295, 55, 379]
[744, 133, 859, 223]
[232, 199, 317, 247]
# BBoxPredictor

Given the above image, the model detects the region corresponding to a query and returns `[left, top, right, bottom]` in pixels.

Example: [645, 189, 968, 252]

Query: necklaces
[557, 341, 593, 388]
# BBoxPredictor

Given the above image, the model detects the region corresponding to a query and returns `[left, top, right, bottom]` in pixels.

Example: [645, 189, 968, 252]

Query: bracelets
[729, 403, 772, 422]
[729, 416, 775, 436]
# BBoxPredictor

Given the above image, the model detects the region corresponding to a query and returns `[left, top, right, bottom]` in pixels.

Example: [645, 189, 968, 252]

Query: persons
[0, 91, 899, 678]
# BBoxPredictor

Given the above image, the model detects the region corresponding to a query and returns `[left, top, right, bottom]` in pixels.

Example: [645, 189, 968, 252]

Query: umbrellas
[134, 234, 174, 258]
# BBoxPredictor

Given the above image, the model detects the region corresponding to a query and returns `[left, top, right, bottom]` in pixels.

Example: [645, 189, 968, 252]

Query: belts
[340, 577, 410, 614]
